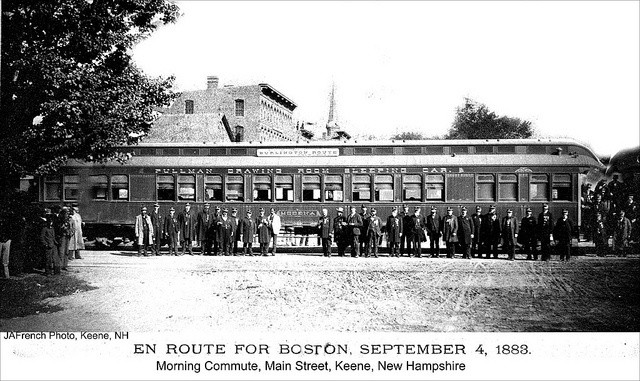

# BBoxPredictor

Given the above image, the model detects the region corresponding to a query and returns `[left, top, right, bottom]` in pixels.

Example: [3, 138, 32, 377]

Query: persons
[501, 209, 518, 259]
[164, 207, 178, 255]
[37, 204, 84, 271]
[387, 207, 403, 257]
[359, 205, 368, 257]
[401, 207, 410, 257]
[471, 206, 482, 258]
[483, 205, 499, 258]
[135, 207, 152, 256]
[553, 208, 573, 260]
[425, 207, 441, 258]
[198, 202, 209, 254]
[411, 207, 424, 257]
[584, 178, 637, 258]
[231, 208, 241, 255]
[539, 203, 552, 260]
[519, 208, 538, 260]
[442, 207, 458, 258]
[242, 210, 254, 256]
[319, 209, 333, 256]
[458, 206, 474, 258]
[221, 210, 230, 256]
[348, 206, 362, 257]
[0, 240, 13, 280]
[334, 208, 347, 256]
[368, 208, 384, 258]
[151, 204, 162, 256]
[256, 208, 269, 255]
[210, 207, 220, 255]
[267, 209, 281, 255]
[179, 203, 194, 255]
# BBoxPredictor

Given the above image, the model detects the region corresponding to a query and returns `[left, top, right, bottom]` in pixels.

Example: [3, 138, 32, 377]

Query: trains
[103, 139, 600, 163]
[17, 155, 608, 249]
